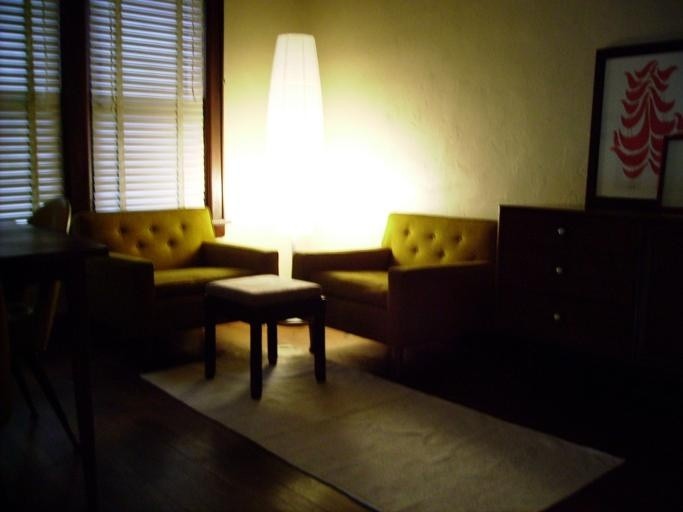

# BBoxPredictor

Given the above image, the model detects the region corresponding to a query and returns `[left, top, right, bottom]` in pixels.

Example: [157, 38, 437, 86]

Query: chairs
[4, 196, 77, 451]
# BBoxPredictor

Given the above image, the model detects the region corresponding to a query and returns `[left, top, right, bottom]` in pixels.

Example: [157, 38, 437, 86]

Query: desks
[0, 219, 109, 478]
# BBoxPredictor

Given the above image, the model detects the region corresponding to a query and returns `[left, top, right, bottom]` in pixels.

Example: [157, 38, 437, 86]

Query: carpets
[139, 344, 624, 512]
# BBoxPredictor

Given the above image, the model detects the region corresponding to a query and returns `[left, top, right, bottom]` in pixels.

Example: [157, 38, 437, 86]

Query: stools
[204, 272, 328, 402]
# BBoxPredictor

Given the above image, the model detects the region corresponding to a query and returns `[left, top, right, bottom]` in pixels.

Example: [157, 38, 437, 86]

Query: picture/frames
[584, 42, 682, 210]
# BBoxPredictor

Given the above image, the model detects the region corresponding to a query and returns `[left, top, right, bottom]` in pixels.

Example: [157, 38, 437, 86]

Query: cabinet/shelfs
[494, 200, 683, 444]
